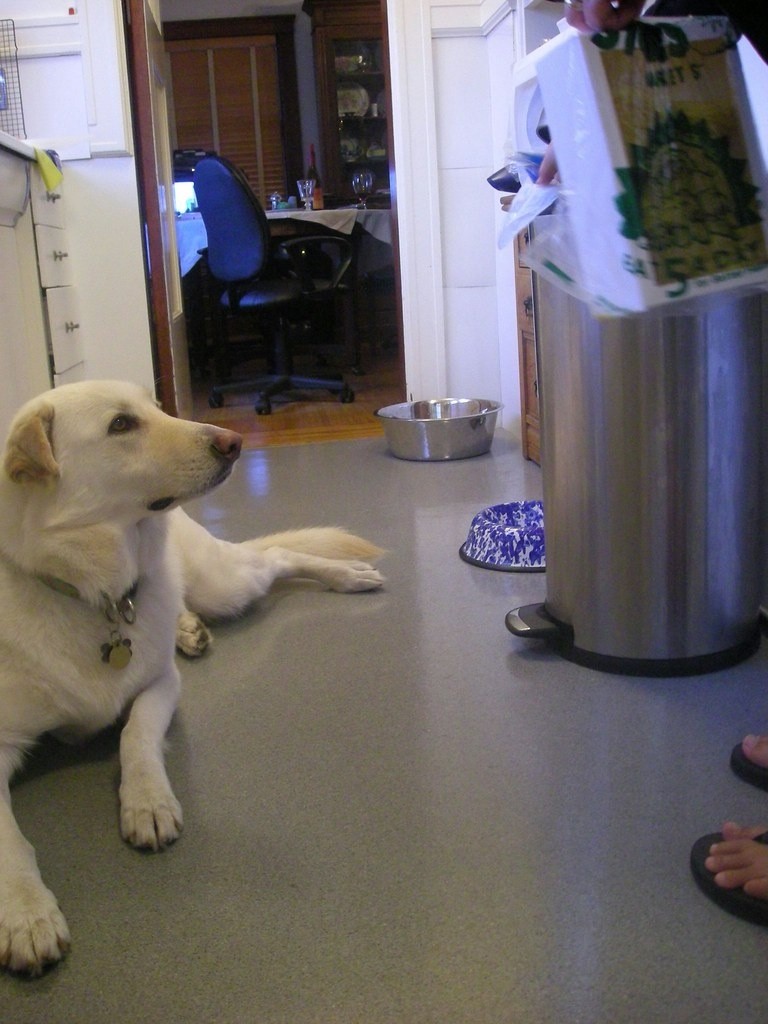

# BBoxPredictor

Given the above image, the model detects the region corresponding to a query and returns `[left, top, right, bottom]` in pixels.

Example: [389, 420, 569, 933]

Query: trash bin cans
[504, 198, 768, 676]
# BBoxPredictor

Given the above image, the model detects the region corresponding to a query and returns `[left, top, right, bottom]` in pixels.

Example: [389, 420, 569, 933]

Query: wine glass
[352, 169, 373, 210]
[296, 179, 317, 210]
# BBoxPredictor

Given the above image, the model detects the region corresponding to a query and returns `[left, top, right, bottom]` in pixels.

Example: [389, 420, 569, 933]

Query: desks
[175, 203, 394, 376]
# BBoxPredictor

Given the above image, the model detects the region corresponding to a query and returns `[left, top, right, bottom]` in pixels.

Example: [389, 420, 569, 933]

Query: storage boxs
[533, 32, 768, 314]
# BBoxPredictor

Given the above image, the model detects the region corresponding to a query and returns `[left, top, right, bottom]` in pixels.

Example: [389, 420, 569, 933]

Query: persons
[537, 0, 768, 926]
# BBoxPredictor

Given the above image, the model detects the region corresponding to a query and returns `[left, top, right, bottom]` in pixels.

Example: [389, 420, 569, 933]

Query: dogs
[1, 376, 387, 981]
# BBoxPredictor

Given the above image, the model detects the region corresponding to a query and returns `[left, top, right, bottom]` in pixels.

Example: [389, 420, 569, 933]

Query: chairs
[193, 154, 357, 417]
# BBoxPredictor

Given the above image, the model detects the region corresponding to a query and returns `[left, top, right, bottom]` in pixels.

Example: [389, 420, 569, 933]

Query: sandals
[730, 734, 768, 793]
[690, 822, 768, 927]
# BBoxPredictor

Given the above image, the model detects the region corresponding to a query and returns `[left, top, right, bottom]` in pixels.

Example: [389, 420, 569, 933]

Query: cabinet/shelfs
[302, 0, 392, 210]
[499, 193, 543, 466]
[0, 139, 95, 463]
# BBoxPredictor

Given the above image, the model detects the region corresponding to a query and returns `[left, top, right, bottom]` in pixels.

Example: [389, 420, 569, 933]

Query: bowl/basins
[374, 398, 506, 463]
